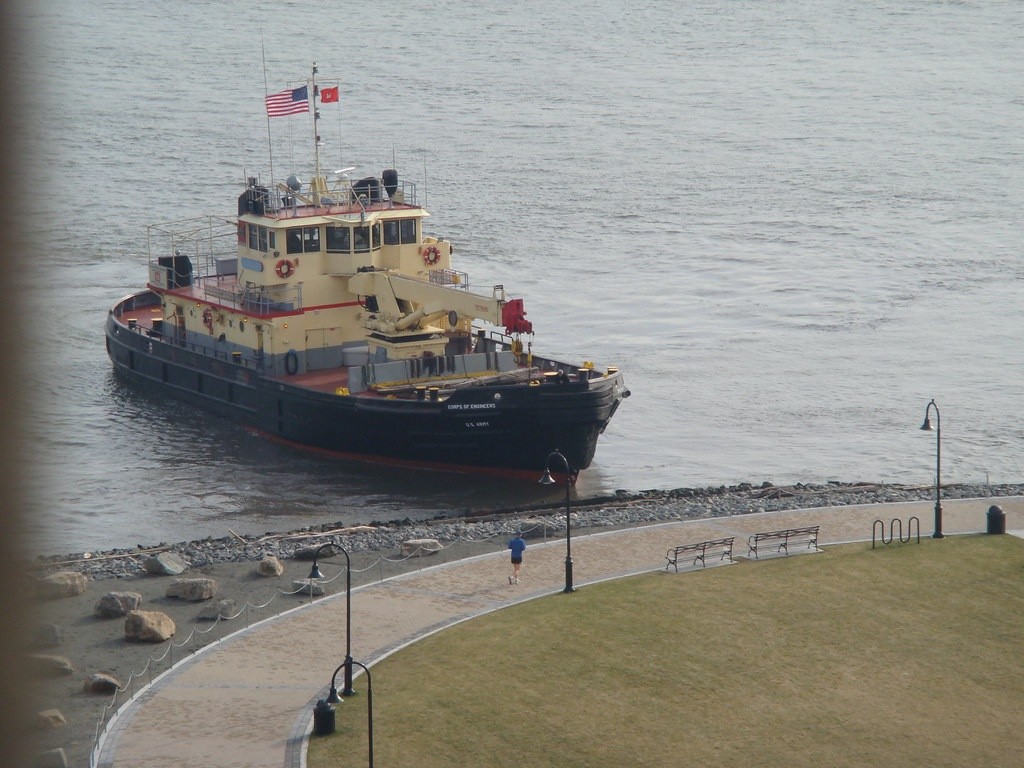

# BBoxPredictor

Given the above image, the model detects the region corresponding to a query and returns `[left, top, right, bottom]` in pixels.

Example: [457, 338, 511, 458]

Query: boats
[105, 62, 631, 487]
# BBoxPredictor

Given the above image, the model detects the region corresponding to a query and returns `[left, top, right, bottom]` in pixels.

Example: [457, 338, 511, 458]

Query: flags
[265, 85, 310, 116]
[320, 86, 339, 103]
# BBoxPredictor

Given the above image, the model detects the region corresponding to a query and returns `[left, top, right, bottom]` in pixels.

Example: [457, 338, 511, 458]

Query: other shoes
[508, 576, 513, 584]
[515, 578, 521, 584]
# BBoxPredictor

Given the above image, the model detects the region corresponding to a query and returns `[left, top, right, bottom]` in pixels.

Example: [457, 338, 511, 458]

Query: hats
[516, 531, 521, 536]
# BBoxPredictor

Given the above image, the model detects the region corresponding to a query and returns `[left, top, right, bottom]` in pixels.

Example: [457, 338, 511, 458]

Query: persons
[508, 531, 526, 585]
[554, 369, 572, 384]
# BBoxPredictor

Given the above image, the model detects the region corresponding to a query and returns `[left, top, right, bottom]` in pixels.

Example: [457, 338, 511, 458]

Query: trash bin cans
[985, 504, 1007, 536]
[314, 702, 336, 735]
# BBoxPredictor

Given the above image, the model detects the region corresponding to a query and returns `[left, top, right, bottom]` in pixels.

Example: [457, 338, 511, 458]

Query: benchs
[747, 525, 820, 559]
[664, 536, 736, 572]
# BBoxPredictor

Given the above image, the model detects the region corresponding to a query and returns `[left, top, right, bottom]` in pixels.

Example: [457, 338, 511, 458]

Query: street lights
[537, 449, 576, 593]
[919, 399, 943, 538]
[325, 662, 373, 768]
[306, 541, 356, 696]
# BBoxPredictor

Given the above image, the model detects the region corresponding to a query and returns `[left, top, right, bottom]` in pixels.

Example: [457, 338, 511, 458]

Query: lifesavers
[275, 258, 295, 279]
[423, 246, 442, 265]
[201, 308, 213, 327]
